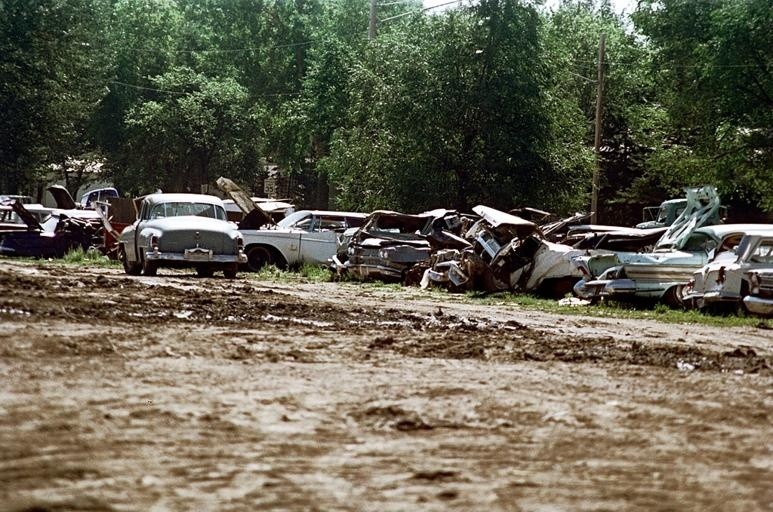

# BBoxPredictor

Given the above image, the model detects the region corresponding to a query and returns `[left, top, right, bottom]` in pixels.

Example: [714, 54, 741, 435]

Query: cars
[0, 177, 295, 279]
